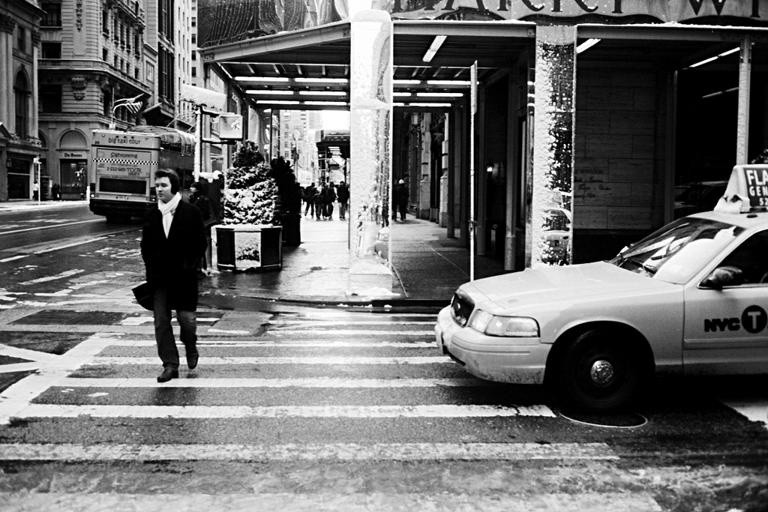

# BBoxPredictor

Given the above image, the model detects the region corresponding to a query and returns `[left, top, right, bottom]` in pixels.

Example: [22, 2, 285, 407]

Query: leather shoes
[158, 368, 178, 382]
[185, 343, 199, 368]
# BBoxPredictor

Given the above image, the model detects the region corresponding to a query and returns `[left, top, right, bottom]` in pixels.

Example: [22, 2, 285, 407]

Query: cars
[434, 212, 768, 407]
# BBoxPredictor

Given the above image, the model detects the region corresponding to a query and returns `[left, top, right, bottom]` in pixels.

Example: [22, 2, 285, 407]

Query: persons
[141, 169, 208, 382]
[298, 181, 351, 220]
[393, 178, 409, 219]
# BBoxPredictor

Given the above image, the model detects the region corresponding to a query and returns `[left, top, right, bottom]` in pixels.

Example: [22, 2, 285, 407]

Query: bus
[90, 129, 221, 220]
